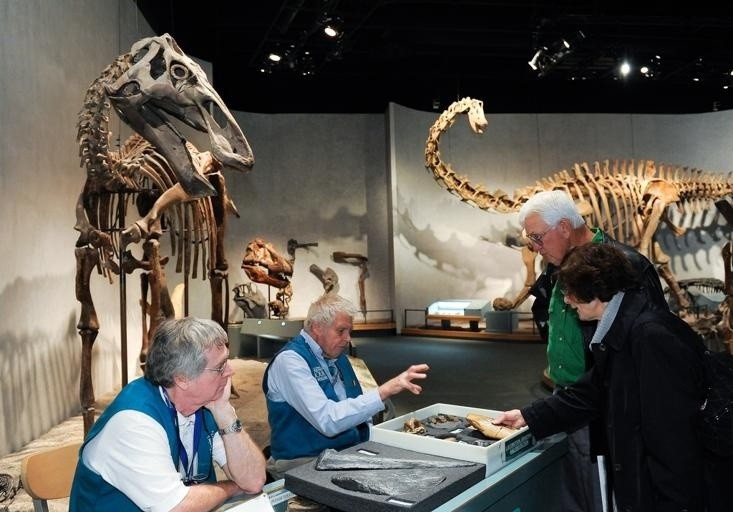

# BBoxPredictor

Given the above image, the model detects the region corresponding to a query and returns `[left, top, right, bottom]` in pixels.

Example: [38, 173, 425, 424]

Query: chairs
[21, 443, 84, 511]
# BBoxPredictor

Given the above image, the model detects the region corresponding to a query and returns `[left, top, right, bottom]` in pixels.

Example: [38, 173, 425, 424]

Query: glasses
[524, 224, 553, 245]
[200, 358, 227, 375]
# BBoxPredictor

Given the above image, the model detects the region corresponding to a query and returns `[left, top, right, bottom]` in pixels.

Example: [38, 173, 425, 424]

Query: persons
[515, 187, 674, 512]
[489, 240, 733, 512]
[258, 293, 431, 473]
[66, 315, 268, 511]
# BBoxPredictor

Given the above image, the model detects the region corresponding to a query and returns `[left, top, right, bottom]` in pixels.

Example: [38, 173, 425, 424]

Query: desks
[220, 435, 581, 510]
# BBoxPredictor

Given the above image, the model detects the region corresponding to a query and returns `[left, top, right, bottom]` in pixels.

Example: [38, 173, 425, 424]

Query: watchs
[216, 417, 242, 435]
